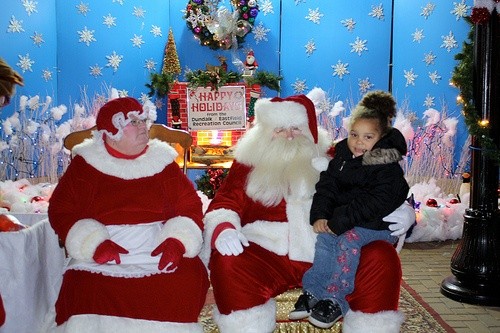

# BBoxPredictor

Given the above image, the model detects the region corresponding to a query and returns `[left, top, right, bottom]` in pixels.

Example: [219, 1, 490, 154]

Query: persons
[288, 90, 417, 329]
[47, 97, 210, 333]
[201, 94, 418, 333]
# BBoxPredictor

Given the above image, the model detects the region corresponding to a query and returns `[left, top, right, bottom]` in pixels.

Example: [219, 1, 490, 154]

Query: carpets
[197, 275, 456, 333]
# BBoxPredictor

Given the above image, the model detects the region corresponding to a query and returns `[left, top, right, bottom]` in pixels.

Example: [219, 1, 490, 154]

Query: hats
[89, 97, 158, 145]
[254, 94, 329, 171]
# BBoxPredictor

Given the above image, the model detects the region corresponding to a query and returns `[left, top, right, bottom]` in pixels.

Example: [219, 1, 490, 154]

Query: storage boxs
[0, 211, 67, 333]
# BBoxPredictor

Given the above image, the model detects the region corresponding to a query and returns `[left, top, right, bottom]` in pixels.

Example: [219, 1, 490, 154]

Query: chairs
[64, 124, 193, 176]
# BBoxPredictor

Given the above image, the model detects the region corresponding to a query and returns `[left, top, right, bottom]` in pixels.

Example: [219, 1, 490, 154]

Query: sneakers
[308, 300, 342, 329]
[289, 293, 318, 319]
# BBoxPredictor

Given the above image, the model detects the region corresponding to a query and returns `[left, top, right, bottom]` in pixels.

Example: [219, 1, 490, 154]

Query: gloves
[382, 204, 416, 236]
[92, 239, 130, 266]
[214, 228, 250, 257]
[150, 237, 186, 271]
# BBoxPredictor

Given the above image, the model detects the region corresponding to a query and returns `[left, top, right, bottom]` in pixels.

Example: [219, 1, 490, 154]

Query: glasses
[126, 119, 147, 127]
[274, 128, 301, 136]
